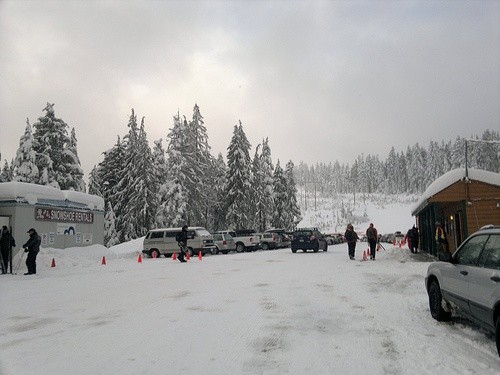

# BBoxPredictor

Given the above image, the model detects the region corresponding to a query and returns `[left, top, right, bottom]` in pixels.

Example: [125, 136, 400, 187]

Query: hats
[349, 226, 354, 229]
[27, 228, 36, 233]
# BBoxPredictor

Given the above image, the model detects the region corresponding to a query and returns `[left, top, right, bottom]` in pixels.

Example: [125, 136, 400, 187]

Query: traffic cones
[102, 256, 106, 264]
[152, 251, 156, 259]
[198, 250, 202, 260]
[173, 252, 177, 260]
[51, 257, 55, 267]
[186, 251, 190, 259]
[362, 251, 367, 261]
[138, 254, 142, 262]
[367, 248, 370, 255]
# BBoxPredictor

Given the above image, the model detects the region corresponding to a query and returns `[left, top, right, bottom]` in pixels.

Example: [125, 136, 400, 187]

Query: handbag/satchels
[181, 246, 189, 253]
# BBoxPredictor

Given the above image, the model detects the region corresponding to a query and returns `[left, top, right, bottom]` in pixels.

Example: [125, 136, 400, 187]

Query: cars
[322, 233, 347, 245]
[377, 231, 404, 244]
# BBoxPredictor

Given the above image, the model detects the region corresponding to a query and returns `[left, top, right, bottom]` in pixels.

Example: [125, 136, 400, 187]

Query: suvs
[210, 233, 236, 255]
[249, 228, 294, 251]
[425, 224, 500, 357]
[291, 228, 328, 253]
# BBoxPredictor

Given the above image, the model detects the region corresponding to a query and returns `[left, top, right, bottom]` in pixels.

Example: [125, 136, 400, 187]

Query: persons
[434, 222, 447, 259]
[177, 225, 191, 262]
[23, 228, 41, 275]
[366, 223, 378, 260]
[344, 223, 359, 260]
[405, 226, 419, 253]
[0, 225, 15, 274]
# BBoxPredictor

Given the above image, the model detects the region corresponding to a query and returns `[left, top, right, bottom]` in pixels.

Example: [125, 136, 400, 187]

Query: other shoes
[177, 256, 182, 261]
[181, 260, 187, 262]
[24, 273, 36, 275]
[370, 255, 372, 259]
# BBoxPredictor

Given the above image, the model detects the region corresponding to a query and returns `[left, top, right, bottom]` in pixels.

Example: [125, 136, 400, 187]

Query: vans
[142, 226, 217, 258]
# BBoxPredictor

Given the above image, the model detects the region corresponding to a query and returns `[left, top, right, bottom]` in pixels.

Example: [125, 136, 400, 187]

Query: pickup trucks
[214, 230, 261, 253]
